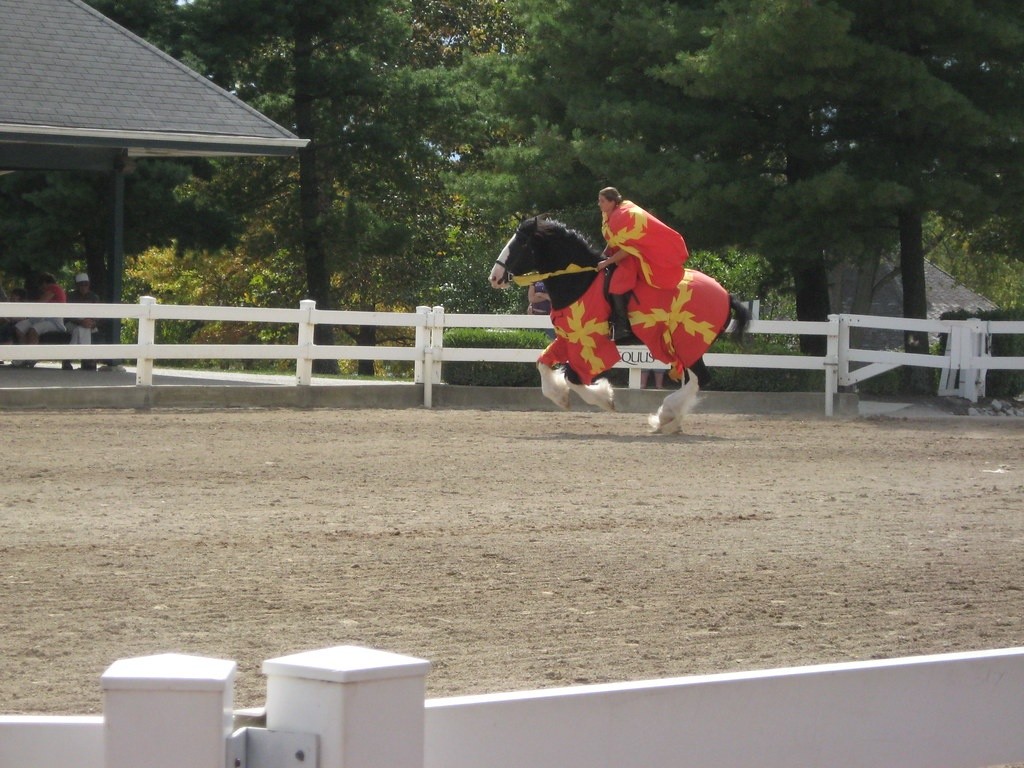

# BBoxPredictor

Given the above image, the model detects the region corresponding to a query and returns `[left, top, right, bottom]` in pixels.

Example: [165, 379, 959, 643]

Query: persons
[530, 281, 553, 340]
[65, 274, 100, 365]
[597, 187, 689, 342]
[15, 274, 67, 344]
[0, 288, 25, 341]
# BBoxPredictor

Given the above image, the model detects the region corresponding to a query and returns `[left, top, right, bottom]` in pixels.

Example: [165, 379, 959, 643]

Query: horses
[488, 215, 749, 437]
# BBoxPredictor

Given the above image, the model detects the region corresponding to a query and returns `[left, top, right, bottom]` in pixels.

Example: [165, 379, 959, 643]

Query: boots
[608, 293, 630, 340]
